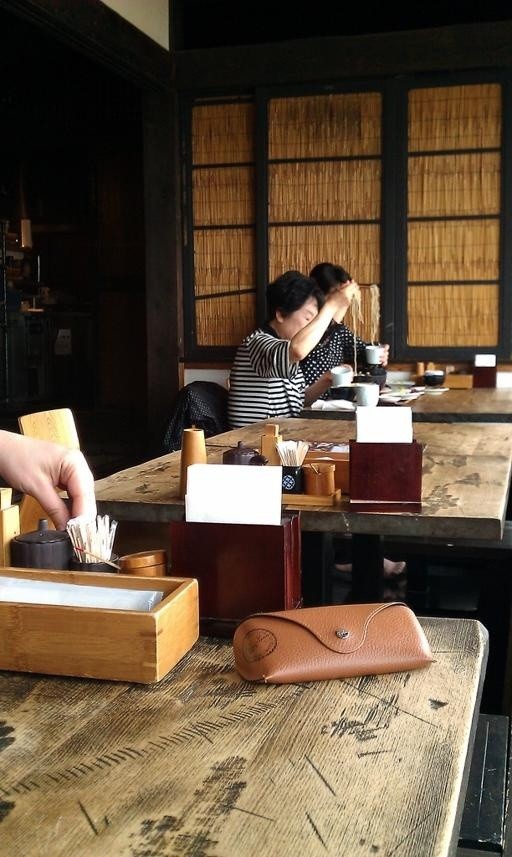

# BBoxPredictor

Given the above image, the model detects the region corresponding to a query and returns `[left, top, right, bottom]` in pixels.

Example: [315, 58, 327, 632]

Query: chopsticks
[278, 440, 309, 467]
[65, 513, 117, 562]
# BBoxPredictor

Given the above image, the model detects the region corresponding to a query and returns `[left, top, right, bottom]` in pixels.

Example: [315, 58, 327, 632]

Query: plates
[379, 387, 391, 394]
[381, 380, 449, 405]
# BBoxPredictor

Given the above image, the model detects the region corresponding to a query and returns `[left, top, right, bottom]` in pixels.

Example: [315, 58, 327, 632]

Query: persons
[228, 262, 389, 429]
[0, 429, 99, 533]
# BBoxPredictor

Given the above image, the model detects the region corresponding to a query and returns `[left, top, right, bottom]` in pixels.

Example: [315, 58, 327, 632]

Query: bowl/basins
[353, 374, 386, 389]
[329, 386, 355, 401]
[423, 374, 446, 388]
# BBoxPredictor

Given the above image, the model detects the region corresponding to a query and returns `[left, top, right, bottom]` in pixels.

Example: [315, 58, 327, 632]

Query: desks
[1, 615, 490, 857]
[49, 412, 512, 590]
[289, 374, 511, 418]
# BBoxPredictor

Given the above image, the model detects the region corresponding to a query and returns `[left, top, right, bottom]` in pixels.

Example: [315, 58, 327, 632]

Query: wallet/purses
[233, 600, 437, 685]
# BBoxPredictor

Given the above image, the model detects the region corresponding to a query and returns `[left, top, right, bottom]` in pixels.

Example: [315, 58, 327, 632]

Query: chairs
[14, 409, 85, 549]
[175, 364, 231, 446]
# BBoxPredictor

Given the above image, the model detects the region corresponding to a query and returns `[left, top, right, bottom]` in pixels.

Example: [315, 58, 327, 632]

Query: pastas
[349, 290, 364, 377]
[370, 284, 381, 346]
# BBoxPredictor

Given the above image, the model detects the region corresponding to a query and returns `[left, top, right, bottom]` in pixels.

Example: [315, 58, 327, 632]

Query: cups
[364, 345, 387, 365]
[282, 463, 334, 496]
[223, 442, 269, 467]
[330, 366, 354, 389]
[354, 383, 380, 408]
[9, 519, 72, 568]
